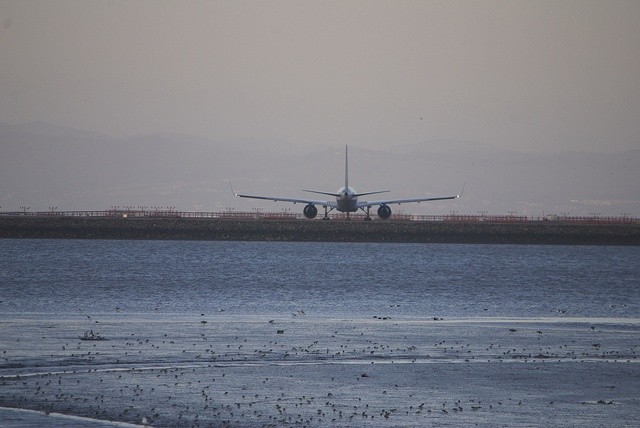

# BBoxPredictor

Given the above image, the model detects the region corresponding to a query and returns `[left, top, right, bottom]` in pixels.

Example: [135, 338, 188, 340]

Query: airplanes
[232, 145, 465, 220]
